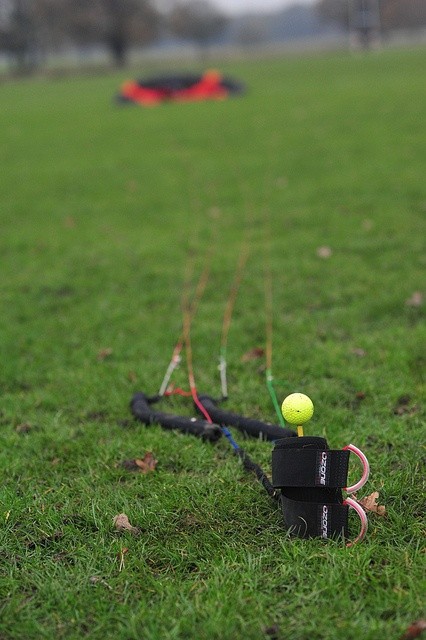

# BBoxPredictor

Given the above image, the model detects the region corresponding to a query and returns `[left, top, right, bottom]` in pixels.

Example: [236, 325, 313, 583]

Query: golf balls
[282, 393, 313, 424]
